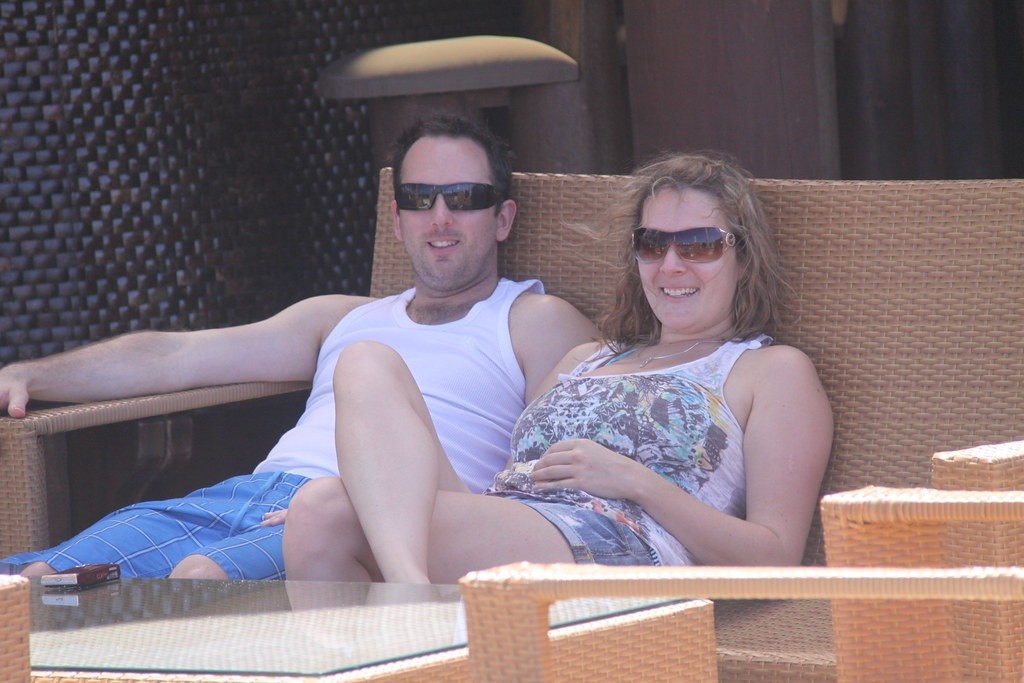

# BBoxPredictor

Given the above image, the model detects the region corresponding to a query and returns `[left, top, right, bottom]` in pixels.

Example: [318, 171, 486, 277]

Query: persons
[0, 112, 606, 578]
[284, 150, 835, 614]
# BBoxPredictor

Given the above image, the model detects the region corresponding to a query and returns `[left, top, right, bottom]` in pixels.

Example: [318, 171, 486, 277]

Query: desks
[28, 578, 686, 683]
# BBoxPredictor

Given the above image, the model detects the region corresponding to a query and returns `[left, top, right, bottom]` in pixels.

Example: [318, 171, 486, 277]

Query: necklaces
[640, 340, 722, 369]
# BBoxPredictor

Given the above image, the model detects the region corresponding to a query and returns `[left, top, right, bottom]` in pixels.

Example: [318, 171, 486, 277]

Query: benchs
[0, 166, 1024, 682]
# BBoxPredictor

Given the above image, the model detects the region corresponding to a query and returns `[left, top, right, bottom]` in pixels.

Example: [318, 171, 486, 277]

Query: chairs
[462, 488, 1024, 683]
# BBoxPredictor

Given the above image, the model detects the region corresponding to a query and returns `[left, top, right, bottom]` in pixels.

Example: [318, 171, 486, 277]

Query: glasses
[395, 183, 508, 211]
[633, 226, 736, 263]
